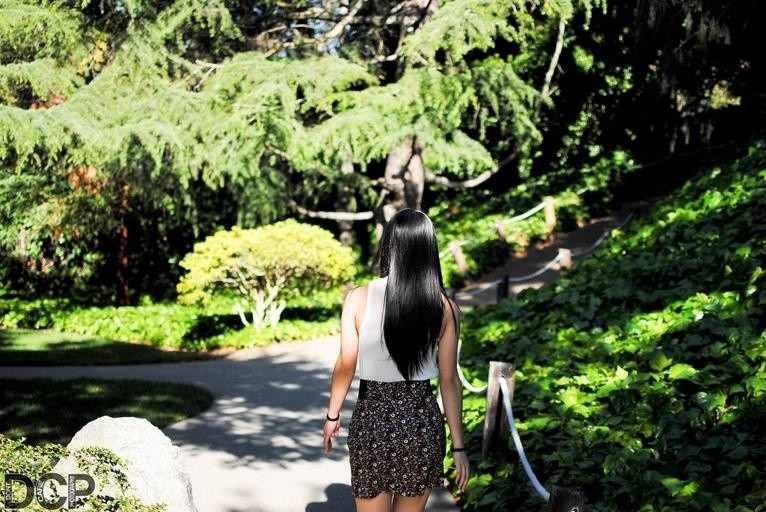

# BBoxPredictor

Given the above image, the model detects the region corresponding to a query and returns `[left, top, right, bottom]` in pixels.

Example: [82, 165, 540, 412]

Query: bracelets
[326, 413, 340, 421]
[452, 447, 466, 452]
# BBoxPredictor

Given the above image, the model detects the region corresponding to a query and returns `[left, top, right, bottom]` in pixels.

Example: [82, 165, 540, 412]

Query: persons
[323, 208, 471, 512]
